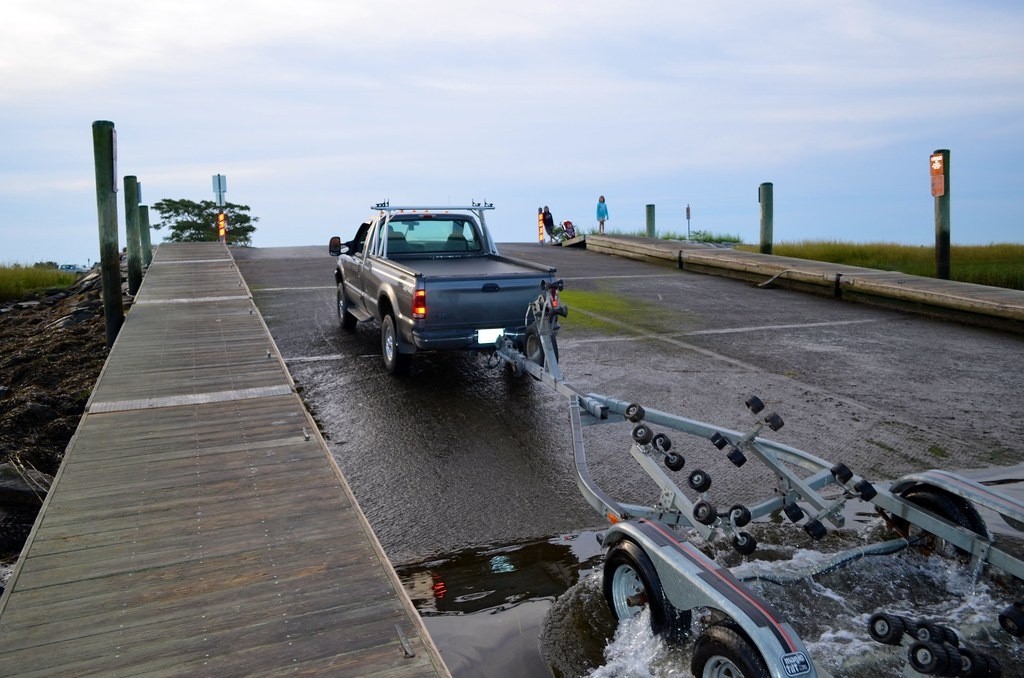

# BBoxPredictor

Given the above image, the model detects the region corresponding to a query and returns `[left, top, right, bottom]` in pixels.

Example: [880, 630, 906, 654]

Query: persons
[330, 239, 340, 252]
[379, 223, 393, 239]
[596, 196, 609, 235]
[543, 206, 558, 244]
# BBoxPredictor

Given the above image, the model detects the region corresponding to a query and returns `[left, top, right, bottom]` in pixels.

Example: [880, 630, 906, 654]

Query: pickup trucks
[328, 198, 560, 380]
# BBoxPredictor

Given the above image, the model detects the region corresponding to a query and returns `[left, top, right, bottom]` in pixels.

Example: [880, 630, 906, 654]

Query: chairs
[441, 232, 469, 250]
[387, 231, 409, 252]
